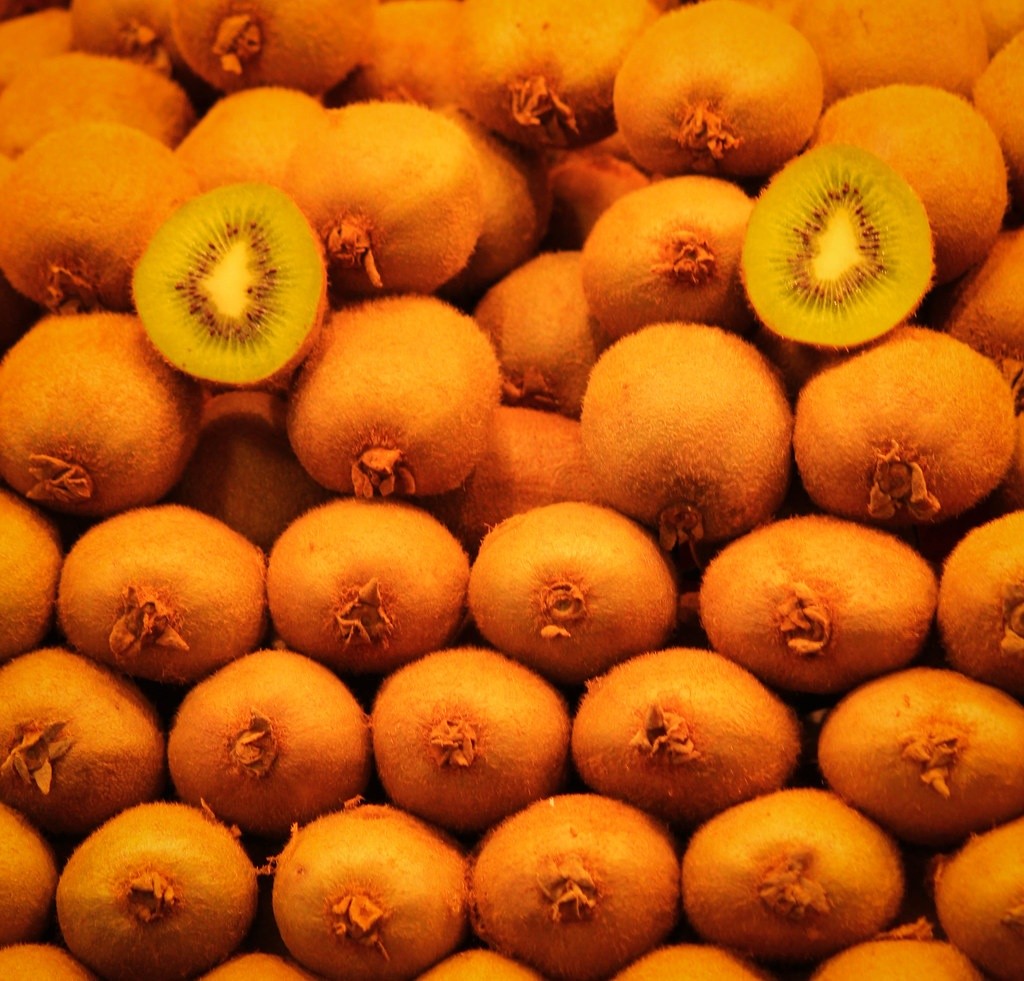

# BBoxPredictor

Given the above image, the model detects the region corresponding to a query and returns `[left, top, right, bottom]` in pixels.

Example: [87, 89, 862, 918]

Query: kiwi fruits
[1, 1, 1024, 981]
